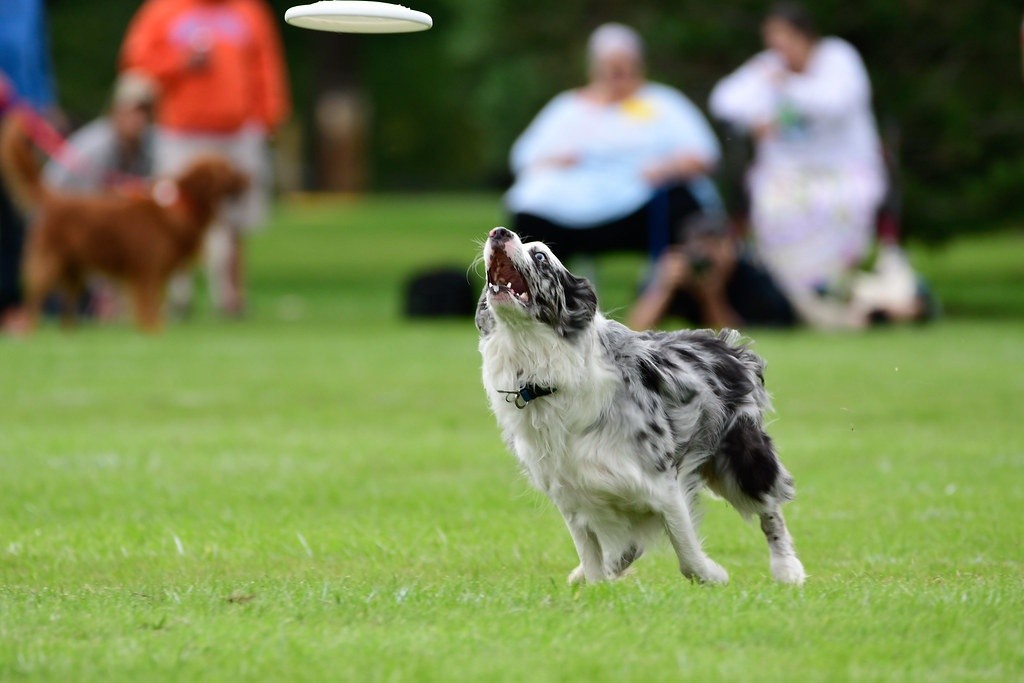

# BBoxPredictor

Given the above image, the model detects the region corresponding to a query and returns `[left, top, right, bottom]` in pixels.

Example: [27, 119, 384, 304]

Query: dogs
[464, 226, 813, 590]
[2, 109, 253, 337]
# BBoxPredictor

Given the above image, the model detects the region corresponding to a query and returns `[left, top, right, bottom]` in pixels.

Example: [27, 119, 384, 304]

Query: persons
[626, 238, 933, 333]
[707, 0, 888, 294]
[39, 83, 152, 317]
[0, 0, 70, 334]
[504, 23, 722, 333]
[118, 0, 289, 318]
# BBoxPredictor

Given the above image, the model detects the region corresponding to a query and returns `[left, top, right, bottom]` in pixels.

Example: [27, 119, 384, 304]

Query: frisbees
[285, 1, 433, 33]
